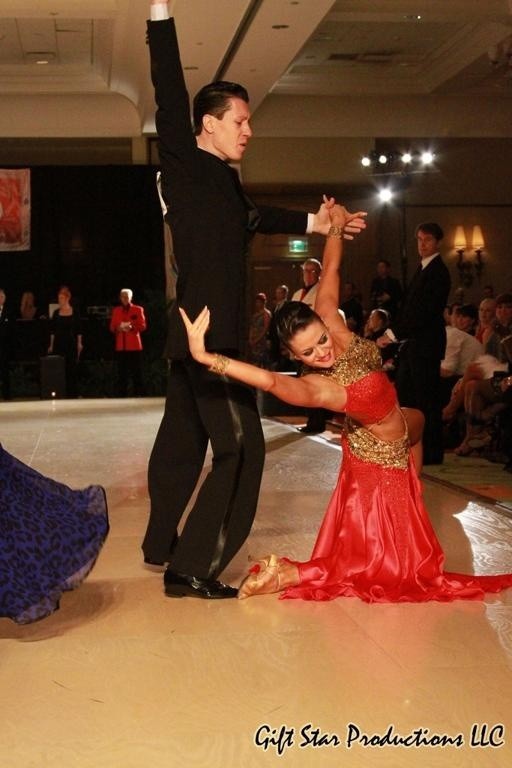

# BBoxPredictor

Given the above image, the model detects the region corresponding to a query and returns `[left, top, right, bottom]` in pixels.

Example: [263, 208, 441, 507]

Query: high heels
[237, 553, 286, 600]
[454, 431, 492, 457]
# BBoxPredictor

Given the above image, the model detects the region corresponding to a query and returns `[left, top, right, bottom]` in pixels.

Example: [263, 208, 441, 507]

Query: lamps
[454, 225, 474, 284]
[471, 224, 488, 282]
[360, 150, 395, 168]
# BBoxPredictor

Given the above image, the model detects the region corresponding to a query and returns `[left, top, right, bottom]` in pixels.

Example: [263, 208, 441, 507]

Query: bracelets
[206, 351, 232, 376]
[326, 224, 345, 240]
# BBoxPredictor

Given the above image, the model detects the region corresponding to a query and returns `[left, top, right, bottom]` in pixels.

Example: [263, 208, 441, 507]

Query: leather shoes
[163, 570, 238, 599]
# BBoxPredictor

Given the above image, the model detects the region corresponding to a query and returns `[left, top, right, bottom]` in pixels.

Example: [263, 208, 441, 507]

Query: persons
[0, 291, 17, 321]
[249, 254, 512, 472]
[111, 287, 146, 397]
[49, 286, 86, 399]
[376, 220, 452, 467]
[17, 291, 47, 321]
[141, 1, 368, 601]
[176, 191, 511, 602]
